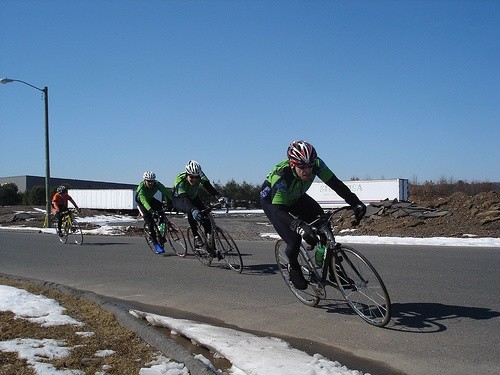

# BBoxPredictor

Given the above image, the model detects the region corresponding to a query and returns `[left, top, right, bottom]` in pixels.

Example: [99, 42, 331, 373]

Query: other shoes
[154, 243, 165, 254]
[329, 273, 355, 290]
[288, 266, 309, 290]
[57, 230, 63, 238]
[195, 236, 204, 247]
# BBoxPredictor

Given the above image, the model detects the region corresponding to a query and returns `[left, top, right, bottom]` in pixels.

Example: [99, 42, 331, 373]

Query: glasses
[188, 175, 201, 179]
[295, 163, 313, 169]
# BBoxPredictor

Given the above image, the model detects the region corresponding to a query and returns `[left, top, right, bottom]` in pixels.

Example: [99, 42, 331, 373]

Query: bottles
[207, 231, 211, 245]
[160, 223, 164, 236]
[306, 246, 319, 267]
[316, 243, 325, 266]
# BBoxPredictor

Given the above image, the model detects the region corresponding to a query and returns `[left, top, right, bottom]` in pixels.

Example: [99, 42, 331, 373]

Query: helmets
[143, 170, 156, 181]
[185, 160, 202, 176]
[57, 185, 67, 194]
[287, 139, 318, 167]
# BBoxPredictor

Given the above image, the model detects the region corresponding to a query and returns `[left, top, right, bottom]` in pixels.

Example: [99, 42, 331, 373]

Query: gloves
[290, 218, 319, 246]
[191, 207, 202, 221]
[345, 193, 368, 220]
[151, 211, 159, 219]
[218, 196, 230, 206]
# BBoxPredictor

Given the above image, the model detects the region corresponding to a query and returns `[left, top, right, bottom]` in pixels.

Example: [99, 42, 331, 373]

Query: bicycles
[54, 208, 84, 246]
[186, 199, 244, 273]
[143, 209, 188, 258]
[275, 207, 393, 328]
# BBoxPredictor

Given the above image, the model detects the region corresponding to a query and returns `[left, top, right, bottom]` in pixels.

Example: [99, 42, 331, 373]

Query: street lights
[0, 77, 53, 227]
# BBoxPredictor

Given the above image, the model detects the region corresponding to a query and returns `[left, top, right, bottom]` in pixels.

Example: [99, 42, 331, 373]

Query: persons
[260, 140, 366, 291]
[135, 171, 172, 253]
[50, 186, 80, 237]
[172, 160, 227, 247]
